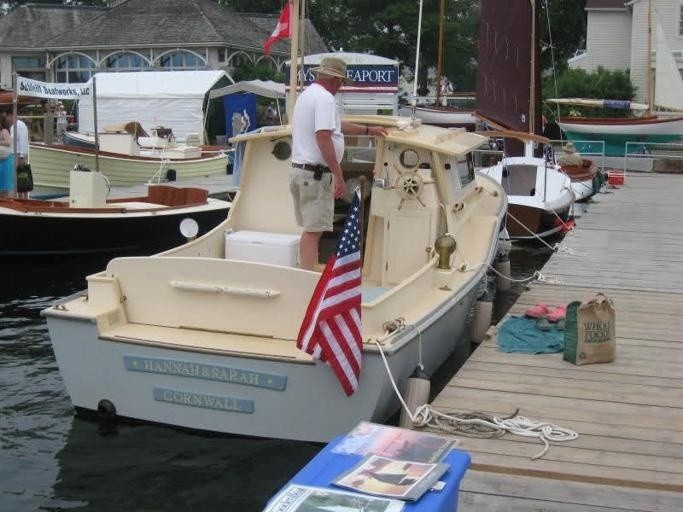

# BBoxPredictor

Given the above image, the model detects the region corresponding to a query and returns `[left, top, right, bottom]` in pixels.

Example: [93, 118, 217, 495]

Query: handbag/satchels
[563, 293, 616, 366]
[17, 164, 33, 193]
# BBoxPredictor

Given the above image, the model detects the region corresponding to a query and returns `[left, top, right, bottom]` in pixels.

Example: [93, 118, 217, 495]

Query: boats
[554, 115, 683, 160]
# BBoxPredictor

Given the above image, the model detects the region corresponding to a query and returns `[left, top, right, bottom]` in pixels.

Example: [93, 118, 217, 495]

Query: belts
[292, 164, 331, 173]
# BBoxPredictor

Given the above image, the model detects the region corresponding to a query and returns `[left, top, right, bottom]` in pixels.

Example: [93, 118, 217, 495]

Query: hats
[309, 56, 354, 84]
[562, 143, 576, 153]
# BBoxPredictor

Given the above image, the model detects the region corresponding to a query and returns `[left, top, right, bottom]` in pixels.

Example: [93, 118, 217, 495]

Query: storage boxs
[224, 228, 301, 266]
[267, 432, 471, 512]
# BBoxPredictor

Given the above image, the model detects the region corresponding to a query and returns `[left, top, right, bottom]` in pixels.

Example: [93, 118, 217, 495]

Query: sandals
[526, 304, 567, 331]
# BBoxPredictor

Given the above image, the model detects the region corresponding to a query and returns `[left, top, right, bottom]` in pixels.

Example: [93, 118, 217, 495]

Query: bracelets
[367, 128, 368, 135]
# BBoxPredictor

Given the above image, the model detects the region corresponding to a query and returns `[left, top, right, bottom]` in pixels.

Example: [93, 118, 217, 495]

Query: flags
[263, 3, 289, 56]
[297, 185, 364, 402]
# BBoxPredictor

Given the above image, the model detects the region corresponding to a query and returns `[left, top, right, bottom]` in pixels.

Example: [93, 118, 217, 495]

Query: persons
[558, 143, 583, 169]
[6, 109, 32, 201]
[54, 101, 68, 143]
[289, 55, 388, 270]
[0, 109, 11, 200]
